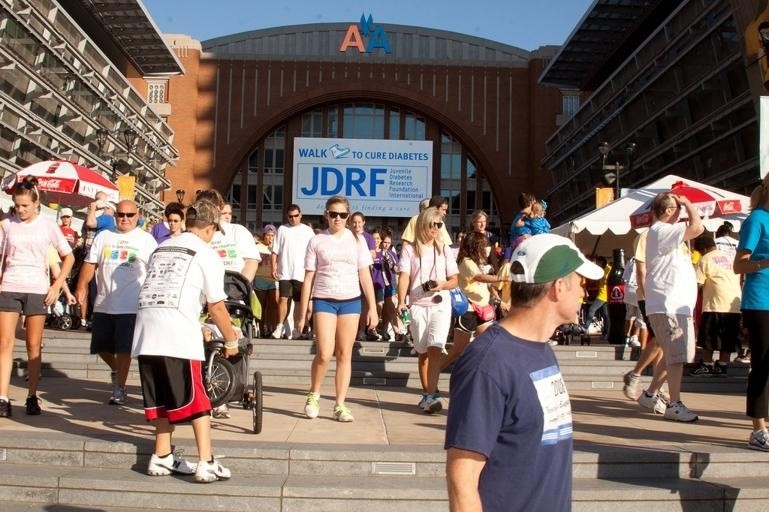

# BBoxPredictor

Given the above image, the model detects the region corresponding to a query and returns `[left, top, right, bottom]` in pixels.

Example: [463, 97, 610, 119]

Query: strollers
[44, 233, 97, 331]
[557, 321, 592, 346]
[199, 268, 264, 435]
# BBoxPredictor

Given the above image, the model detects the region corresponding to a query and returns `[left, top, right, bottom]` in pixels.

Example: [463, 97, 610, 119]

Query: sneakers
[2, 370, 232, 482]
[620, 334, 769, 452]
[270, 322, 448, 420]
[547, 339, 558, 346]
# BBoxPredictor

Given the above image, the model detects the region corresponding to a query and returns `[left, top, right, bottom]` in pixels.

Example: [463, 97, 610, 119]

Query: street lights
[175, 188, 186, 205]
[94, 125, 139, 181]
[597, 138, 638, 201]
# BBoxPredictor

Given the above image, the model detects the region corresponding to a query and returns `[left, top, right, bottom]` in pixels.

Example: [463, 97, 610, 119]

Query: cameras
[489, 294, 501, 305]
[422, 280, 437, 293]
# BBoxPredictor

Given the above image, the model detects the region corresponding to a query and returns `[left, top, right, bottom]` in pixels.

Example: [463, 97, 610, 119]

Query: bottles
[401, 309, 412, 326]
[605, 248, 629, 345]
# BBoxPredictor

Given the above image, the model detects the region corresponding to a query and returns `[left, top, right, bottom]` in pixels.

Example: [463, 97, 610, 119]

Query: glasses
[288, 214, 299, 218]
[116, 211, 136, 218]
[326, 210, 349, 219]
[430, 222, 444, 229]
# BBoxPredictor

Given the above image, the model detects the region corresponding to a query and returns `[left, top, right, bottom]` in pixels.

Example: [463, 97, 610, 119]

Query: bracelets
[224, 341, 238, 348]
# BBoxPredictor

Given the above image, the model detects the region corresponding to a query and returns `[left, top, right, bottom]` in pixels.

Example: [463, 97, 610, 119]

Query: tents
[549, 176, 752, 252]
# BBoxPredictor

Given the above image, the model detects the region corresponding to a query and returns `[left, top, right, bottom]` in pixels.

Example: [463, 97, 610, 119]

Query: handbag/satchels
[450, 284, 468, 317]
[471, 303, 496, 321]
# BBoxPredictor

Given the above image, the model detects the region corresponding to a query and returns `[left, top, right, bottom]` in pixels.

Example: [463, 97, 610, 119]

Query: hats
[509, 232, 606, 285]
[186, 200, 226, 236]
[263, 224, 276, 235]
[59, 208, 74, 219]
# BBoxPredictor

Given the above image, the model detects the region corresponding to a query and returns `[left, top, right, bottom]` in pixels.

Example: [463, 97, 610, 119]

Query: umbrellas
[0, 155, 122, 231]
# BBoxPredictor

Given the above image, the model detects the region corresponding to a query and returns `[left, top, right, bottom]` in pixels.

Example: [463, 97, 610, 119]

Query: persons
[444, 234, 604, 512]
[296, 196, 379, 421]
[147, 202, 185, 243]
[132, 200, 239, 482]
[351, 211, 412, 343]
[624, 192, 748, 422]
[733, 172, 768, 451]
[202, 313, 243, 342]
[75, 200, 159, 404]
[196, 189, 261, 418]
[220, 202, 323, 340]
[0, 176, 76, 417]
[396, 197, 551, 413]
[563, 256, 613, 340]
[9, 193, 116, 327]
